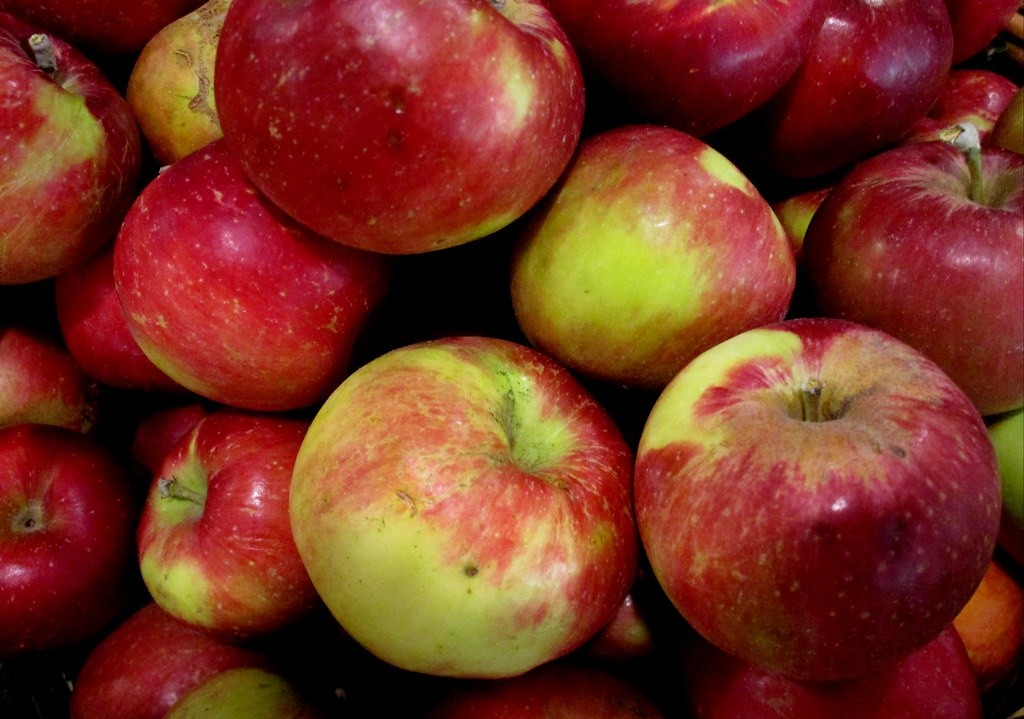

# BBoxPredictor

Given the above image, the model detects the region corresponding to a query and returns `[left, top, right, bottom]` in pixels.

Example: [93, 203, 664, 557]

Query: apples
[0, 0, 1024, 719]
